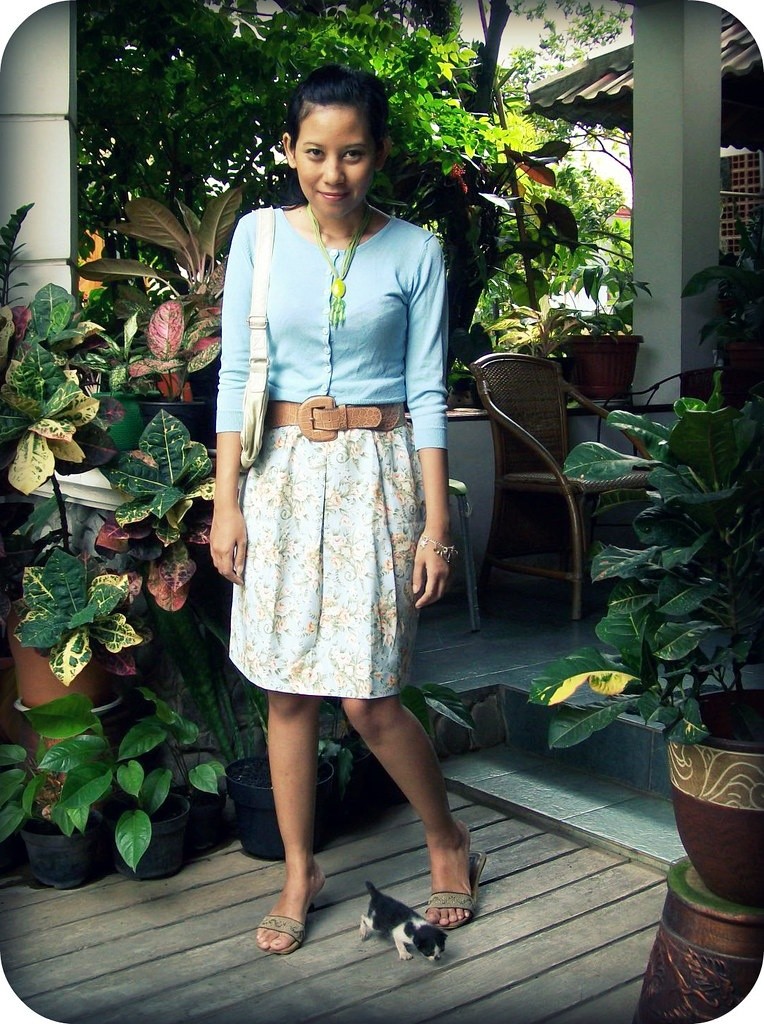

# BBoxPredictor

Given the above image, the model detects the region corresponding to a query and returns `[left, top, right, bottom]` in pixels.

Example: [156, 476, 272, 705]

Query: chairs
[471, 351, 657, 622]
[588, 365, 751, 593]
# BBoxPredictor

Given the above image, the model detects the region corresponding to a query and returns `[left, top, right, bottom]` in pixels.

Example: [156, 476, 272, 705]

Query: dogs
[360, 880, 450, 964]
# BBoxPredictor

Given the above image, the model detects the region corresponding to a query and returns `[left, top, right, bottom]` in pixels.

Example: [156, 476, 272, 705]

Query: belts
[265, 395, 407, 443]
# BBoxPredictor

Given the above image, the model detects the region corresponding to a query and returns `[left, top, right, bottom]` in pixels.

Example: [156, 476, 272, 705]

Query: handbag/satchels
[239, 207, 275, 469]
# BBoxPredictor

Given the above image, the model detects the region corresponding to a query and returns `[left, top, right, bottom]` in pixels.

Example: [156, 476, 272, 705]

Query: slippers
[256, 902, 314, 955]
[425, 850, 487, 930]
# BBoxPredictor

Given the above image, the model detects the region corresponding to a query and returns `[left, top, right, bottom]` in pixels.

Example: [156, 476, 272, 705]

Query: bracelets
[419, 535, 460, 563]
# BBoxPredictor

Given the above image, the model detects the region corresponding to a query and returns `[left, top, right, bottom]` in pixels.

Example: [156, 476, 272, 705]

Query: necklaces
[305, 202, 372, 325]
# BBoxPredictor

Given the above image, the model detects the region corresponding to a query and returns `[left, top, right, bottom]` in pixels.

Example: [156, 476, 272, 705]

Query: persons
[209, 71, 485, 959]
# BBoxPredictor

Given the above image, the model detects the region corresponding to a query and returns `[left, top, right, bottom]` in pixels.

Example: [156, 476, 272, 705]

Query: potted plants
[0, 185, 474, 893]
[545, 266, 654, 400]
[525, 205, 764, 910]
